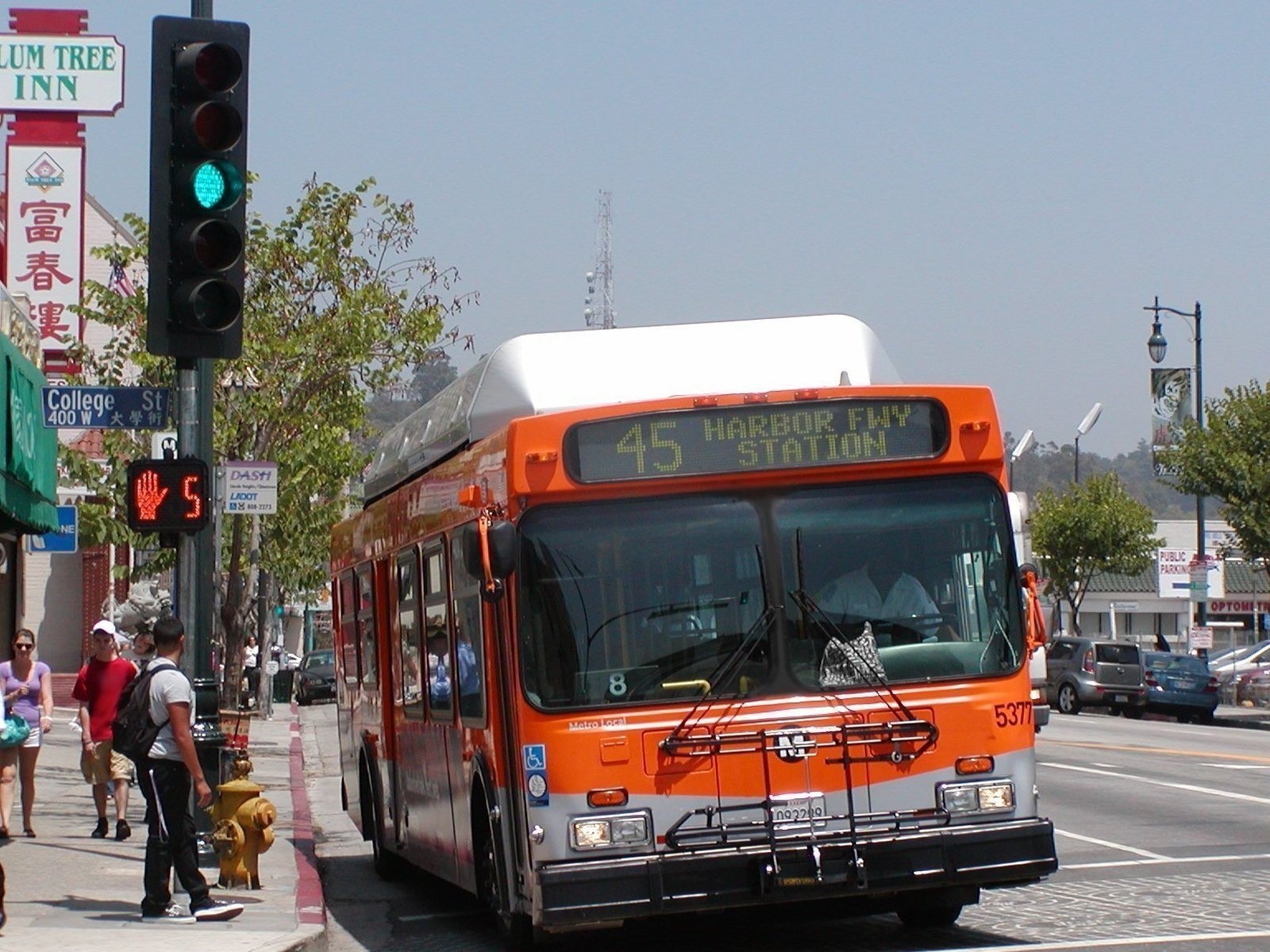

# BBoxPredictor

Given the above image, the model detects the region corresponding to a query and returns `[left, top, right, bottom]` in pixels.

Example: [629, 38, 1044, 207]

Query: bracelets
[40, 716, 52, 722]
[84, 740, 92, 745]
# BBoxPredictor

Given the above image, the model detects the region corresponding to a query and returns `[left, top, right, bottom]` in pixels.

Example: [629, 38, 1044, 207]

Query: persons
[358, 596, 481, 715]
[808, 531, 943, 648]
[0, 615, 258, 938]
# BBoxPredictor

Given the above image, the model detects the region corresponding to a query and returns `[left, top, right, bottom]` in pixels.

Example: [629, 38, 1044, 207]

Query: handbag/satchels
[0, 699, 30, 748]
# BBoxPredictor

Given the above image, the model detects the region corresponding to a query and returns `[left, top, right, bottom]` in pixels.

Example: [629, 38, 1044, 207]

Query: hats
[93, 620, 115, 635]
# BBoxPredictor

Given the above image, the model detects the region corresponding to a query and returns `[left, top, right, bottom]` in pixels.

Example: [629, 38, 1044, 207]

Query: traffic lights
[277, 605, 285, 620]
[145, 15, 255, 363]
[121, 456, 217, 538]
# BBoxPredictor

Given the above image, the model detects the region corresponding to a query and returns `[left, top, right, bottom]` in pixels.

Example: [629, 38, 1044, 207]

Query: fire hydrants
[201, 755, 280, 891]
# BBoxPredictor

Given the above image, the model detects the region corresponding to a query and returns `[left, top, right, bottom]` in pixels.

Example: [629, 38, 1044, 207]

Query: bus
[329, 314, 1062, 952]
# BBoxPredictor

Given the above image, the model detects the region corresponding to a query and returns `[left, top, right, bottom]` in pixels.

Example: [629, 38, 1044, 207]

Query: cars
[1206, 638, 1270, 710]
[1106, 650, 1221, 723]
[1025, 644, 1050, 733]
[293, 649, 335, 705]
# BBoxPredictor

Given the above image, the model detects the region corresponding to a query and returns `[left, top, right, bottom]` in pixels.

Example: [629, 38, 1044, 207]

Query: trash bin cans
[217, 709, 260, 796]
[273, 670, 294, 703]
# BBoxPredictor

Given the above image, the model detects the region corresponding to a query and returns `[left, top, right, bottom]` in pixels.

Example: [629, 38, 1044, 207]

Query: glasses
[14, 643, 34, 650]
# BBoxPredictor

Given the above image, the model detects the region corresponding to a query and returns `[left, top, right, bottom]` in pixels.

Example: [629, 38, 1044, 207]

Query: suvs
[1041, 635, 1146, 716]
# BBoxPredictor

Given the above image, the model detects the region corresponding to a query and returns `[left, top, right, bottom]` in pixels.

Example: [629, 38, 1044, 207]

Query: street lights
[1008, 429, 1038, 496]
[1141, 297, 1207, 670]
[1072, 401, 1105, 492]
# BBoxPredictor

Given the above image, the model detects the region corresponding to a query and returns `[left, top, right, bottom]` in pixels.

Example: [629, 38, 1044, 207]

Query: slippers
[23, 826, 36, 838]
[0, 825, 9, 838]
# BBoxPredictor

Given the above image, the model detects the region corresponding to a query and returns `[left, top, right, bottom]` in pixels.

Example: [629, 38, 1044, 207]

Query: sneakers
[195, 897, 244, 921]
[142, 900, 196, 924]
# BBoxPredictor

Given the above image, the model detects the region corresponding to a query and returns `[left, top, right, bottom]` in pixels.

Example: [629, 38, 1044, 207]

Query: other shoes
[92, 819, 108, 838]
[116, 820, 130, 841]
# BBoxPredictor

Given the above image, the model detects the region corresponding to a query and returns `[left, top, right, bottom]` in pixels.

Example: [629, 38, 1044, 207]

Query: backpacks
[112, 660, 172, 762]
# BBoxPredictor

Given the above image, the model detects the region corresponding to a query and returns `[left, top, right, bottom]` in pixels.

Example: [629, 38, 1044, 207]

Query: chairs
[653, 608, 706, 649]
